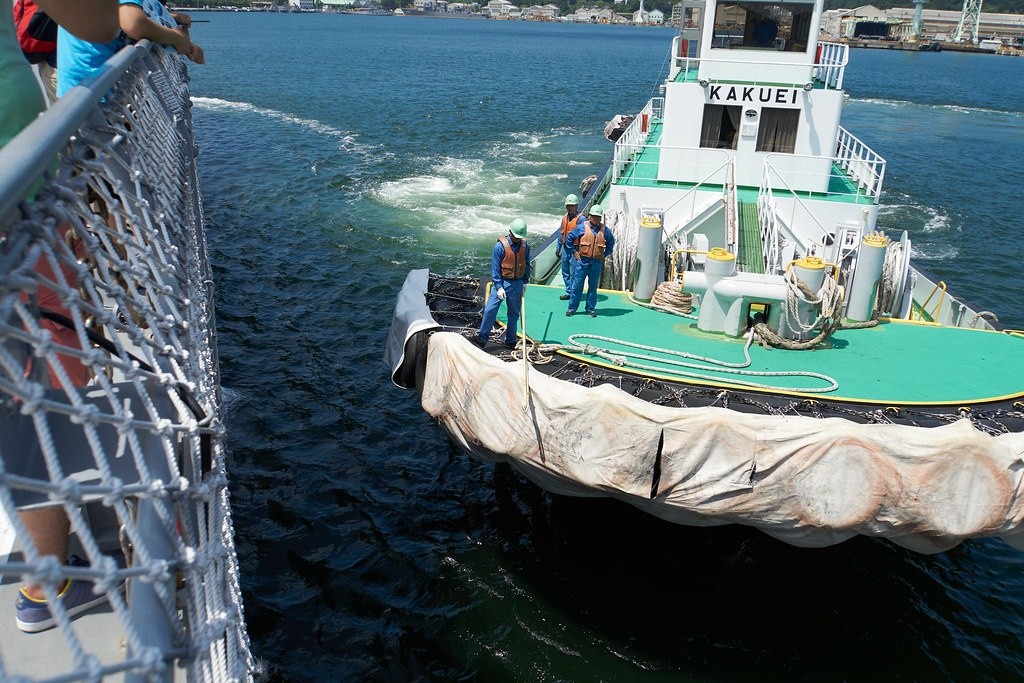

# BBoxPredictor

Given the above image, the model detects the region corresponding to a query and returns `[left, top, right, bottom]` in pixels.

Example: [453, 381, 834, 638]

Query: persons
[566, 205, 614, 316]
[461, 219, 530, 350]
[756, 7, 777, 47]
[0, 0, 204, 634]
[556, 193, 586, 300]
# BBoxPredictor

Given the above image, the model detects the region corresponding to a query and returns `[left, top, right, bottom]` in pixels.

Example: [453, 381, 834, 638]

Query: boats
[388, 0, 1023, 549]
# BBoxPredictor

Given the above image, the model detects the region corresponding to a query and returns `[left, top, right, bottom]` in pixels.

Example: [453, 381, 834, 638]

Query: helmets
[509, 218, 527, 238]
[565, 194, 579, 205]
[588, 205, 604, 216]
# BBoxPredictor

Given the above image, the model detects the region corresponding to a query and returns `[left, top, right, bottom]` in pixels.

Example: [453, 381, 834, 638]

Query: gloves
[523, 284, 527, 292]
[556, 249, 560, 257]
[497, 287, 506, 301]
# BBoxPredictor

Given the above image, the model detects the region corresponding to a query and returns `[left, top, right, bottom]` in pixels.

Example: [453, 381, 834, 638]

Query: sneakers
[15, 554, 127, 632]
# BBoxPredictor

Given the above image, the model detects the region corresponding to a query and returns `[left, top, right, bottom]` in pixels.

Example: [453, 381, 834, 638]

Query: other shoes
[566, 310, 575, 316]
[470, 335, 485, 348]
[587, 308, 596, 317]
[505, 341, 517, 348]
[560, 295, 570, 300]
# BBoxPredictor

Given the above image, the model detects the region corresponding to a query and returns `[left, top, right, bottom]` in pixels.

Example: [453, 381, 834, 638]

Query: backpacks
[13, 0, 57, 64]
